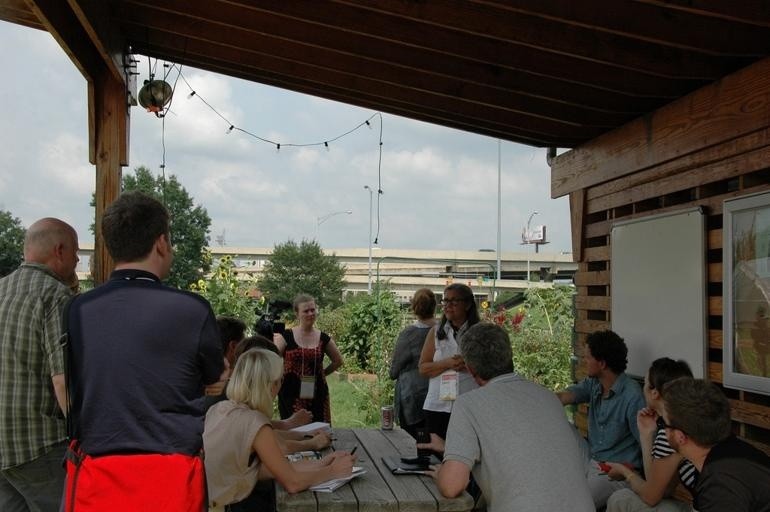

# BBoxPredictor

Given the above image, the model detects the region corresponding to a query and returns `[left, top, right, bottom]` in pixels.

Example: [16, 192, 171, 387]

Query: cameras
[255, 298, 293, 341]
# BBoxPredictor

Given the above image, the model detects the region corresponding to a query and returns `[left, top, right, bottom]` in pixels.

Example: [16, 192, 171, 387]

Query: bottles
[381, 407, 393, 430]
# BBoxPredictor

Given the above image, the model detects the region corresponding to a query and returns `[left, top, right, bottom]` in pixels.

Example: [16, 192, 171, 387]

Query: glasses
[440, 297, 464, 306]
[656, 415, 688, 438]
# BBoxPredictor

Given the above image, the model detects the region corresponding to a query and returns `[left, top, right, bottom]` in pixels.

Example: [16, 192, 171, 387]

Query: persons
[663, 379, 770, 512]
[607, 357, 696, 511]
[555, 330, 645, 508]
[203, 348, 357, 511]
[273, 296, 343, 428]
[389, 289, 437, 433]
[220, 336, 333, 452]
[0, 217, 78, 512]
[63, 193, 224, 511]
[418, 322, 596, 512]
[216, 315, 313, 428]
[418, 282, 481, 436]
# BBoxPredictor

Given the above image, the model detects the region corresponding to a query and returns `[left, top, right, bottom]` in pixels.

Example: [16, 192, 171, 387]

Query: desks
[275, 427, 474, 511]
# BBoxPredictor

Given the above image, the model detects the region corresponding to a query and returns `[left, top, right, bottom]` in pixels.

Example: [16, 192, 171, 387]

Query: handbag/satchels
[59, 293, 210, 512]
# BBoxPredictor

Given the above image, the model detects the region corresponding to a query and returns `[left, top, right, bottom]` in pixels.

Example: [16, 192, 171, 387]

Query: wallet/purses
[598, 461, 635, 472]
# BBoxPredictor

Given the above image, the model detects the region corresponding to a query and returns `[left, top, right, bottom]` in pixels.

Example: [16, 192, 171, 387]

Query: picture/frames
[721, 191, 770, 395]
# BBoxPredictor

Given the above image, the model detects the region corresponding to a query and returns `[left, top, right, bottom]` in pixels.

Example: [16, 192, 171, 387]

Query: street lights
[527, 211, 540, 280]
[315, 210, 353, 244]
[363, 184, 373, 291]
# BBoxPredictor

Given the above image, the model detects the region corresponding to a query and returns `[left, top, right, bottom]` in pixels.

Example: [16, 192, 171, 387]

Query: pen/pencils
[303, 434, 339, 441]
[598, 472, 608, 475]
[350, 443, 359, 457]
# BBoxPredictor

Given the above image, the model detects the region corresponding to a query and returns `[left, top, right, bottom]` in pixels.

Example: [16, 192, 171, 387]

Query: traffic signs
[481, 301, 488, 309]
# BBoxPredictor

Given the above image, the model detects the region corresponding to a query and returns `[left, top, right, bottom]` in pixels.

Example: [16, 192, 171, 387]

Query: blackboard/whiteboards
[608, 205, 708, 381]
